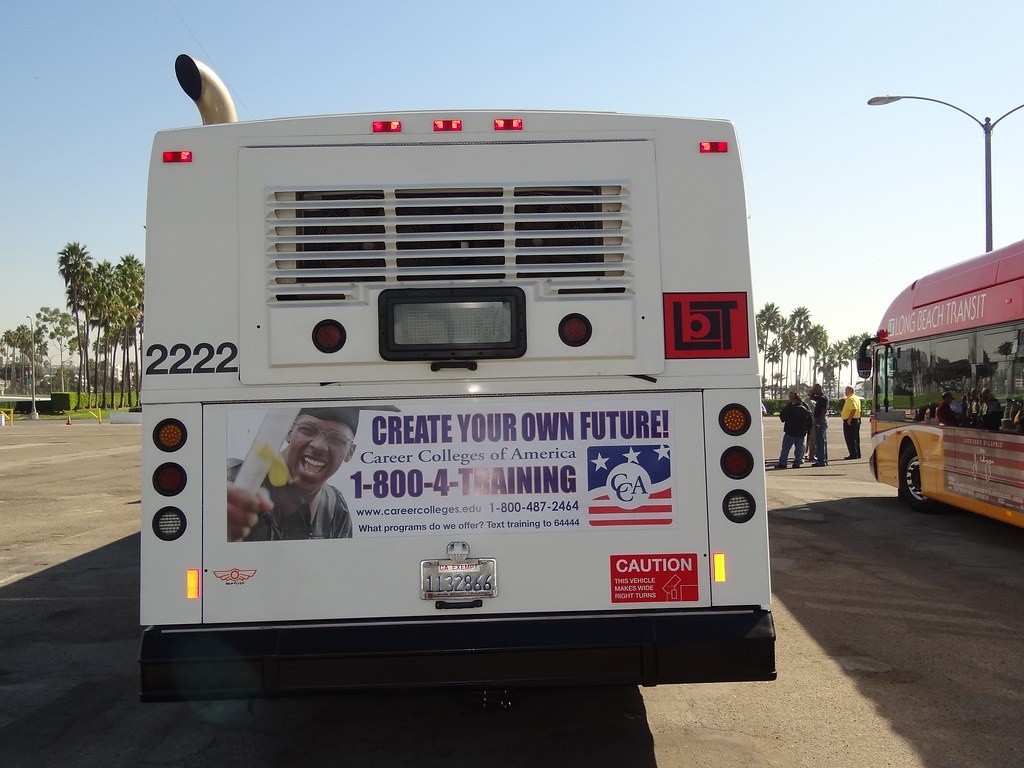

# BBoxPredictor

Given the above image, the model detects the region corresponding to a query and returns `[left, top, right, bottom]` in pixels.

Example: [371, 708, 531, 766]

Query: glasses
[294, 422, 355, 448]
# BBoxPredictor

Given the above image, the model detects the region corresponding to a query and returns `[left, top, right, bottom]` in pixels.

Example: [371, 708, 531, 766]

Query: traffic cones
[66, 416, 73, 425]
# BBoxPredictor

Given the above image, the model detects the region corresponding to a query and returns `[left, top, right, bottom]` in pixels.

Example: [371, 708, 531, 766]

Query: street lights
[867, 96, 1023, 254]
[43, 354, 64, 396]
[26, 315, 39, 420]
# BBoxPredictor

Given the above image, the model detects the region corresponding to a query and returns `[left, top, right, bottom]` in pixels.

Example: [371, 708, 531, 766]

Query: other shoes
[844, 455, 861, 460]
[811, 461, 829, 467]
[774, 464, 787, 469]
[808, 457, 818, 461]
[792, 464, 800, 468]
[801, 459, 805, 464]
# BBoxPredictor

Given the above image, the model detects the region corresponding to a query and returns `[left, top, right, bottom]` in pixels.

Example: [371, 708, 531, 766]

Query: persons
[225, 406, 365, 542]
[841, 386, 862, 460]
[774, 383, 830, 468]
[914, 387, 1023, 434]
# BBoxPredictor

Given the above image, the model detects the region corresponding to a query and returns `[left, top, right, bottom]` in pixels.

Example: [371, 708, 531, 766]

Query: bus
[855, 241, 1024, 531]
[135, 50, 786, 705]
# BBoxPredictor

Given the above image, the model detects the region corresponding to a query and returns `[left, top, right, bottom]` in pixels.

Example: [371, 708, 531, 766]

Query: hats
[298, 405, 401, 438]
